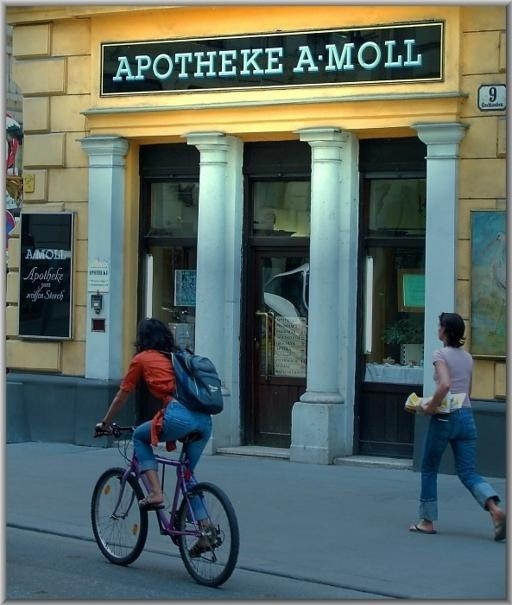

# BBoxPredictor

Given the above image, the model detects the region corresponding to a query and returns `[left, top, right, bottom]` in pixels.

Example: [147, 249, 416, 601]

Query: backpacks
[154, 347, 223, 414]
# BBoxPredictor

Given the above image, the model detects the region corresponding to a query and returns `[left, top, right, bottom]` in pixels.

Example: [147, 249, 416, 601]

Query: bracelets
[102, 422, 111, 428]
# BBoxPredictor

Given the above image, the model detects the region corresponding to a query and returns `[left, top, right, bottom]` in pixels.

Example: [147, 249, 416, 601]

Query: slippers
[138, 496, 166, 510]
[408, 523, 436, 535]
[494, 515, 505, 541]
[189, 536, 222, 555]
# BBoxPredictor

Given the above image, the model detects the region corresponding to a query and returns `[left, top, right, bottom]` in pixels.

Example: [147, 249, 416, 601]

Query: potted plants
[384, 318, 424, 366]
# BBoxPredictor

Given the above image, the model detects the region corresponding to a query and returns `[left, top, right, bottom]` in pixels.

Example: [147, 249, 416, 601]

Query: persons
[94, 315, 224, 558]
[407, 311, 506, 542]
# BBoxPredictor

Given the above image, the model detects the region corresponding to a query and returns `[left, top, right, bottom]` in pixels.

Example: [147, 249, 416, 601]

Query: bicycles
[91, 425, 240, 587]
